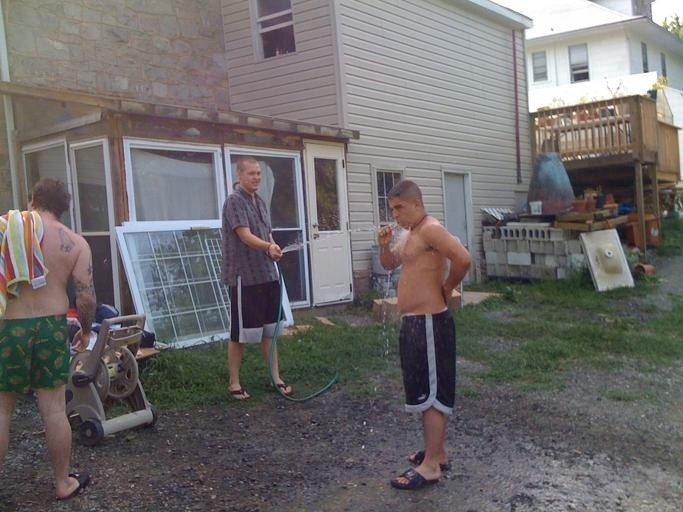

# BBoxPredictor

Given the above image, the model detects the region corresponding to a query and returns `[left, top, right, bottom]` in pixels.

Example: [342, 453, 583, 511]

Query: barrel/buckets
[635, 262, 657, 274]
[530, 201, 543, 216]
[370, 243, 393, 275]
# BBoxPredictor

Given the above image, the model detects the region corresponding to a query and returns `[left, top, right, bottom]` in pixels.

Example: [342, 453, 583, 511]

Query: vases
[586, 200, 597, 212]
[572, 199, 587, 212]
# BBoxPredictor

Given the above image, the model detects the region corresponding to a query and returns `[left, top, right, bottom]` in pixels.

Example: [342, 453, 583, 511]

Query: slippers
[391, 469, 440, 490]
[408, 449, 451, 470]
[272, 379, 292, 395]
[228, 386, 251, 401]
[60, 471, 89, 499]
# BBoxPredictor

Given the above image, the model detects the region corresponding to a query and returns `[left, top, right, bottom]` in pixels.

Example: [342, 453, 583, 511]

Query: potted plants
[632, 263, 656, 284]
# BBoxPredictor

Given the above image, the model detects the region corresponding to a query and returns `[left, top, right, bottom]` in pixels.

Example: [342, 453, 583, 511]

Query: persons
[376, 180, 469, 490]
[221, 155, 293, 400]
[1, 179, 97, 498]
